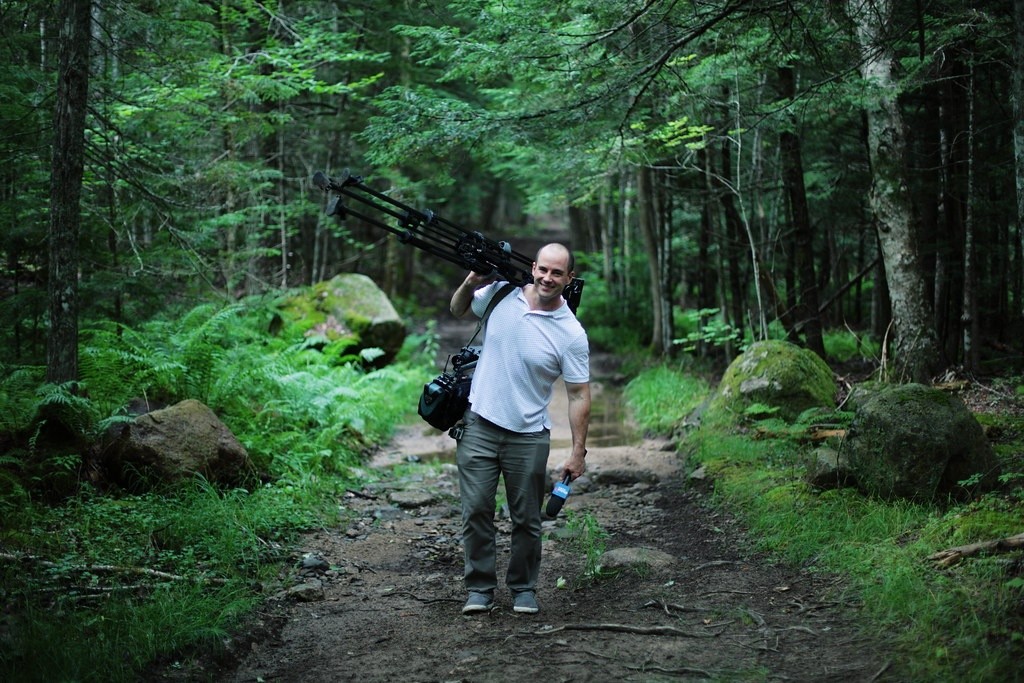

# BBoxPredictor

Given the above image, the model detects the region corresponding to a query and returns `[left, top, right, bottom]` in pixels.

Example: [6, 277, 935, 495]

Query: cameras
[452, 345, 480, 381]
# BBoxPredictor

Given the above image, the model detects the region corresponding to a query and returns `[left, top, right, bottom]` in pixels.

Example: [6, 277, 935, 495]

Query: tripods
[314, 173, 585, 302]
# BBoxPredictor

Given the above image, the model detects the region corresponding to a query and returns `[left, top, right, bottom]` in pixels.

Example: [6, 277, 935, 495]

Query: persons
[447, 240, 592, 614]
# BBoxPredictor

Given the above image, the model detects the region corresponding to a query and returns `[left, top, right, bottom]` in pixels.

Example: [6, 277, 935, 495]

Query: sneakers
[462, 590, 495, 613]
[514, 590, 539, 615]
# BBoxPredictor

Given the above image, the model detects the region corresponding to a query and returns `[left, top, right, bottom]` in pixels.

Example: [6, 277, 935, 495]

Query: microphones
[546, 449, 587, 517]
